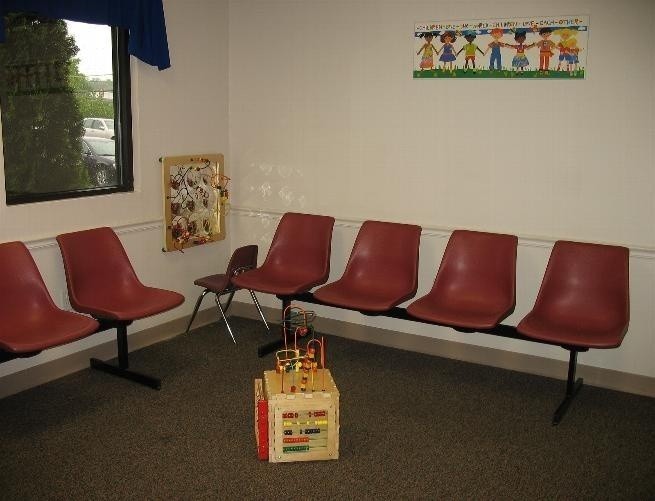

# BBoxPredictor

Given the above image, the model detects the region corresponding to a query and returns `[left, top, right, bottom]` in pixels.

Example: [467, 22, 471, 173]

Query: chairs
[313, 218, 422, 316]
[517, 237, 633, 424]
[229, 211, 338, 359]
[185, 243, 270, 345]
[404, 227, 519, 335]
[0, 224, 185, 393]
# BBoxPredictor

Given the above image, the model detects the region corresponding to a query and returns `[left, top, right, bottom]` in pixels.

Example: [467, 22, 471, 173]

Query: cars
[80, 115, 116, 187]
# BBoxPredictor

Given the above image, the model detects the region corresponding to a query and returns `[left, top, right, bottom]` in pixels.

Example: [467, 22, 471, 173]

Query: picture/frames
[161, 152, 228, 254]
